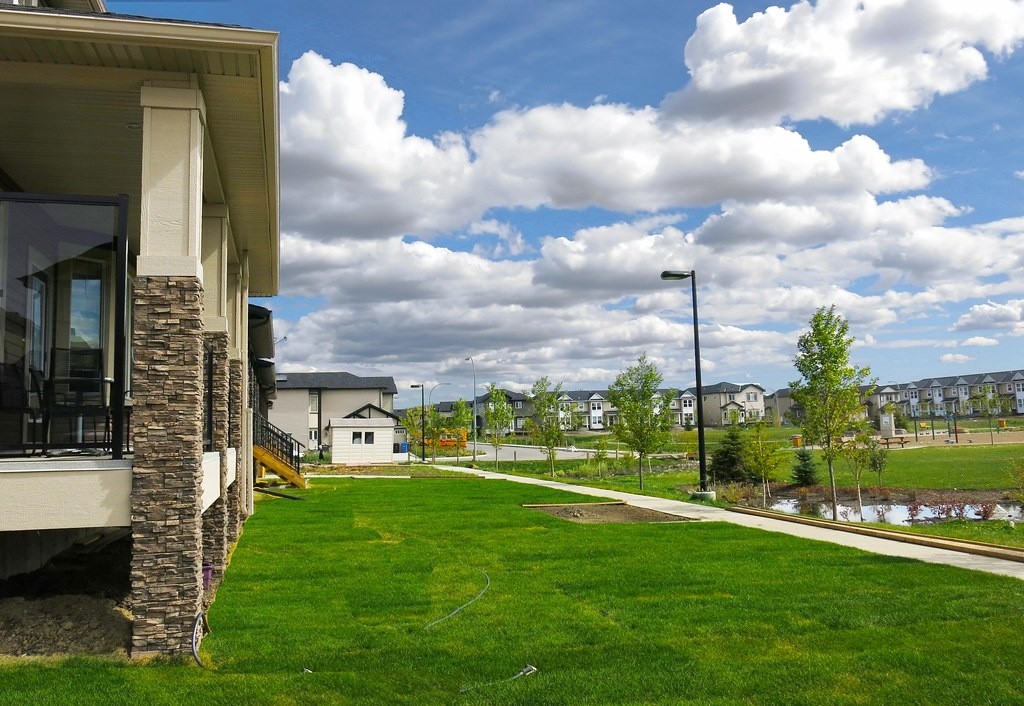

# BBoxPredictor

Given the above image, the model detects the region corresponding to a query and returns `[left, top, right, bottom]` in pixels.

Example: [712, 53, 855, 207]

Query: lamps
[267, 400, 273, 406]
[319, 443, 332, 450]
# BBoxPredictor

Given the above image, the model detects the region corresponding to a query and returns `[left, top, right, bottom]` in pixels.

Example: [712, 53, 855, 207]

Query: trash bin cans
[393, 443, 400, 453]
[401, 443, 407, 453]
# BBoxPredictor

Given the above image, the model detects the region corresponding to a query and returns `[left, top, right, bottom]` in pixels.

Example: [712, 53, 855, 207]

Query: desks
[42, 376, 115, 457]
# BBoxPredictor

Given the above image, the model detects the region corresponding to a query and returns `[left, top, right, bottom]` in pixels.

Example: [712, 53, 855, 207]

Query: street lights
[429, 381, 452, 407]
[660, 269, 709, 490]
[465, 356, 478, 463]
[410, 384, 426, 461]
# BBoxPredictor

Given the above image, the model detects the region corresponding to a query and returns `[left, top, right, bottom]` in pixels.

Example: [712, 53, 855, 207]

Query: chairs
[0, 347, 135, 459]
